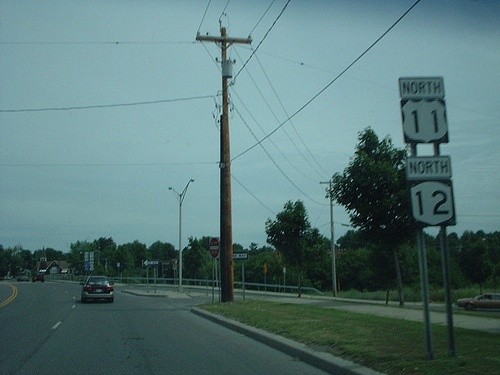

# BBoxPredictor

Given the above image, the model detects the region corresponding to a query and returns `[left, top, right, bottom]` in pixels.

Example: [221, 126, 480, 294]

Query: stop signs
[208, 236, 220, 260]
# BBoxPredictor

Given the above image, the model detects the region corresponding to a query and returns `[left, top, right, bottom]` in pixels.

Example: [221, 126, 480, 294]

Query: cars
[32, 273, 44, 282]
[79, 275, 115, 304]
[16, 275, 29, 282]
[456, 294, 500, 312]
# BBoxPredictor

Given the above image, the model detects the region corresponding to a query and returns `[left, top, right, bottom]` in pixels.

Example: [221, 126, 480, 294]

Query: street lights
[168, 179, 195, 293]
